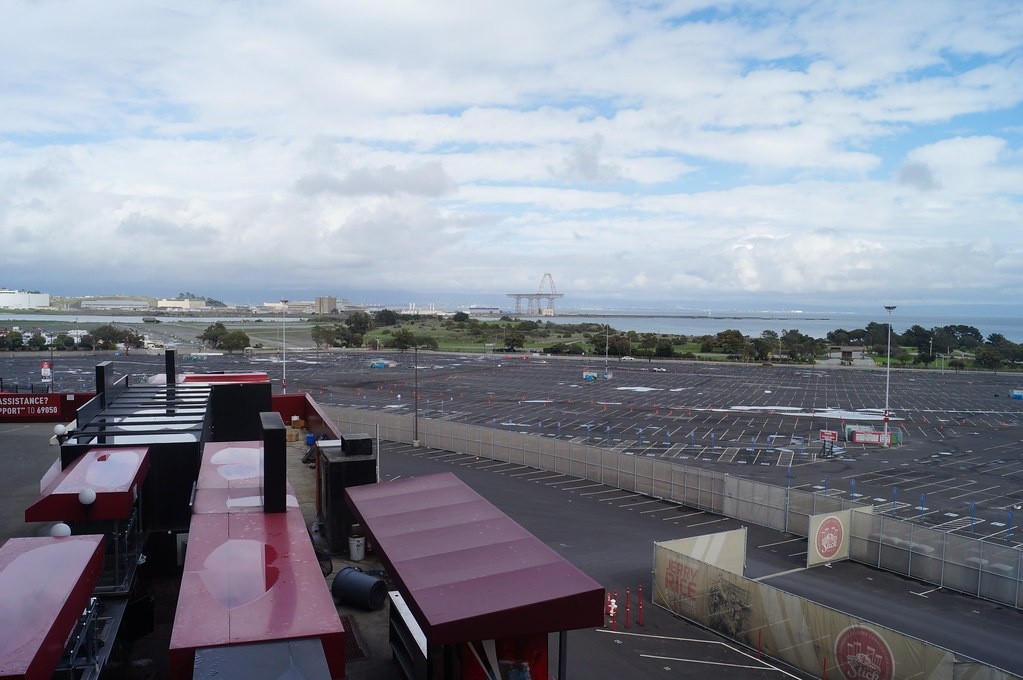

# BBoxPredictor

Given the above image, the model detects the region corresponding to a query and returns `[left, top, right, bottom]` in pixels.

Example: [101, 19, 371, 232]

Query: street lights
[883, 306, 897, 447]
[279, 299, 288, 393]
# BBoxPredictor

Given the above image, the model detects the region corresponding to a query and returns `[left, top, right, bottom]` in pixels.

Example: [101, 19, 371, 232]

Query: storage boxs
[286, 429, 299, 441]
[293, 419, 304, 429]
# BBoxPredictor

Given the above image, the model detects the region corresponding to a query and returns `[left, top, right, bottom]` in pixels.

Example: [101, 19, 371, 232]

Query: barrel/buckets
[349, 534, 365, 561]
[291, 415, 299, 427]
[332, 566, 387, 612]
